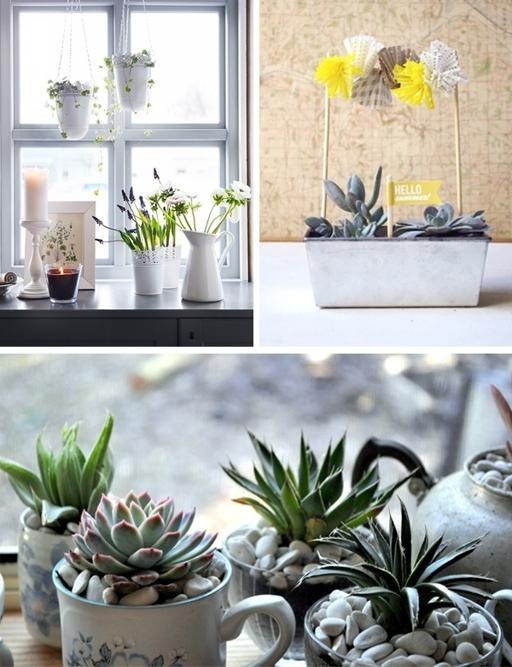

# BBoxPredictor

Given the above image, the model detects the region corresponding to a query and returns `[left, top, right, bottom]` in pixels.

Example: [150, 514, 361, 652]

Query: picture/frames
[24, 200, 97, 291]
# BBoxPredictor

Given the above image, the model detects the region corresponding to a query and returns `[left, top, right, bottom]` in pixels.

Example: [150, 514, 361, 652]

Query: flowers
[91, 184, 161, 250]
[134, 167, 176, 246]
[150, 177, 251, 233]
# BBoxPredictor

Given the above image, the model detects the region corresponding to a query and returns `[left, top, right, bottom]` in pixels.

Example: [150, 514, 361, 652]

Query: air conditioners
[221, 417, 423, 662]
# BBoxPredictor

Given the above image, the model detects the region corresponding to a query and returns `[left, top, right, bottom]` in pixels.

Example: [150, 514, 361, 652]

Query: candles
[22, 170, 48, 221]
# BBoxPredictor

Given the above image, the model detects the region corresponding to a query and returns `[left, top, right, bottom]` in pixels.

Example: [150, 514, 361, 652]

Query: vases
[159, 245, 178, 288]
[130, 249, 163, 296]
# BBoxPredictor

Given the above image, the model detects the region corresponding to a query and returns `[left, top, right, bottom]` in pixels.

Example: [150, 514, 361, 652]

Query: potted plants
[301, 508, 512, 667]
[46, 79, 104, 152]
[298, 164, 492, 307]
[54, 492, 295, 666]
[409, 376, 512, 591]
[95, 43, 158, 142]
[0, 408, 115, 653]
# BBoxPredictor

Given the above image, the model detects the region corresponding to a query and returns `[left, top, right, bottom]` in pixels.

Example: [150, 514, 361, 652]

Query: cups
[44, 262, 83, 304]
[53, 549, 295, 666]
